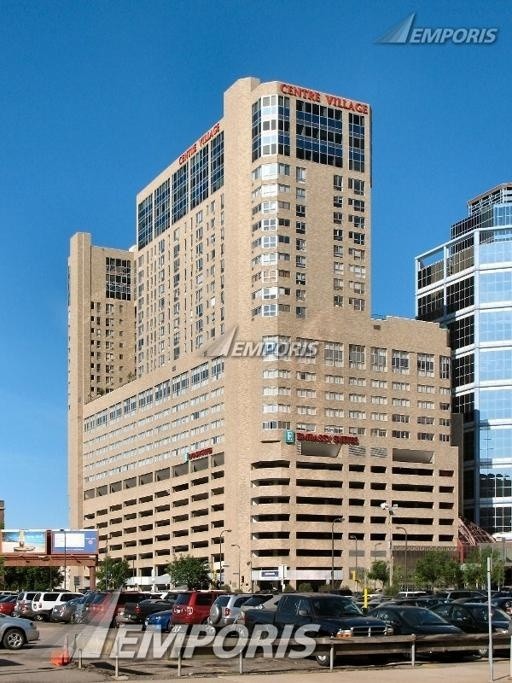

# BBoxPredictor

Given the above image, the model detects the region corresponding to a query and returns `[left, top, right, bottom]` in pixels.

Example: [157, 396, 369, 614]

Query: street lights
[58, 529, 68, 591]
[217, 527, 242, 595]
[330, 516, 408, 589]
